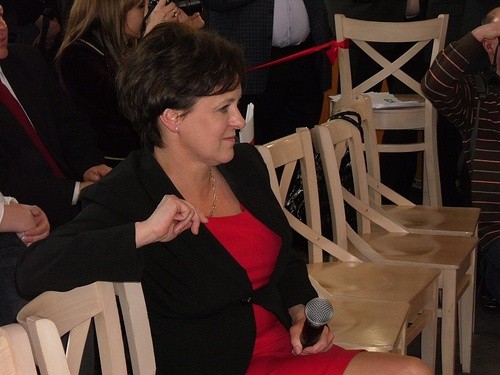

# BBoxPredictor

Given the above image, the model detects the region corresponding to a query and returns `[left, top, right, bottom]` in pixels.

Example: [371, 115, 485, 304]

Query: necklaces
[208, 166, 219, 219]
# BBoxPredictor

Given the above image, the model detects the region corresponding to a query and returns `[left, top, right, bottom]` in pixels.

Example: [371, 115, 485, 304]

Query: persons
[201, 0, 333, 144]
[0, 192, 50, 248]
[0, 5, 113, 218]
[53, 0, 193, 185]
[420, 7, 500, 291]
[15, 0, 62, 49]
[9, 22, 434, 375]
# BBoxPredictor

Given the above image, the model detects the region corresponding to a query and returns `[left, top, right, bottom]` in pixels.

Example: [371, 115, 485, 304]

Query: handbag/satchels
[280, 110, 367, 240]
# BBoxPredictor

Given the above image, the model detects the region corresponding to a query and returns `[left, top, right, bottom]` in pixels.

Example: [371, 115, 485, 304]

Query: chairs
[0, 14, 481, 375]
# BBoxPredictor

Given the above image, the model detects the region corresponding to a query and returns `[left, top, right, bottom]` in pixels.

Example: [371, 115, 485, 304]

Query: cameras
[148, 0, 203, 16]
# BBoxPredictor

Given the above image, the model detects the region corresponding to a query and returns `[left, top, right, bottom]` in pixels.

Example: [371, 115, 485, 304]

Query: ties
[0, 80, 64, 180]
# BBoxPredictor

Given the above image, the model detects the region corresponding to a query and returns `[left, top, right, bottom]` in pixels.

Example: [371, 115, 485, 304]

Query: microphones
[299, 297, 335, 350]
